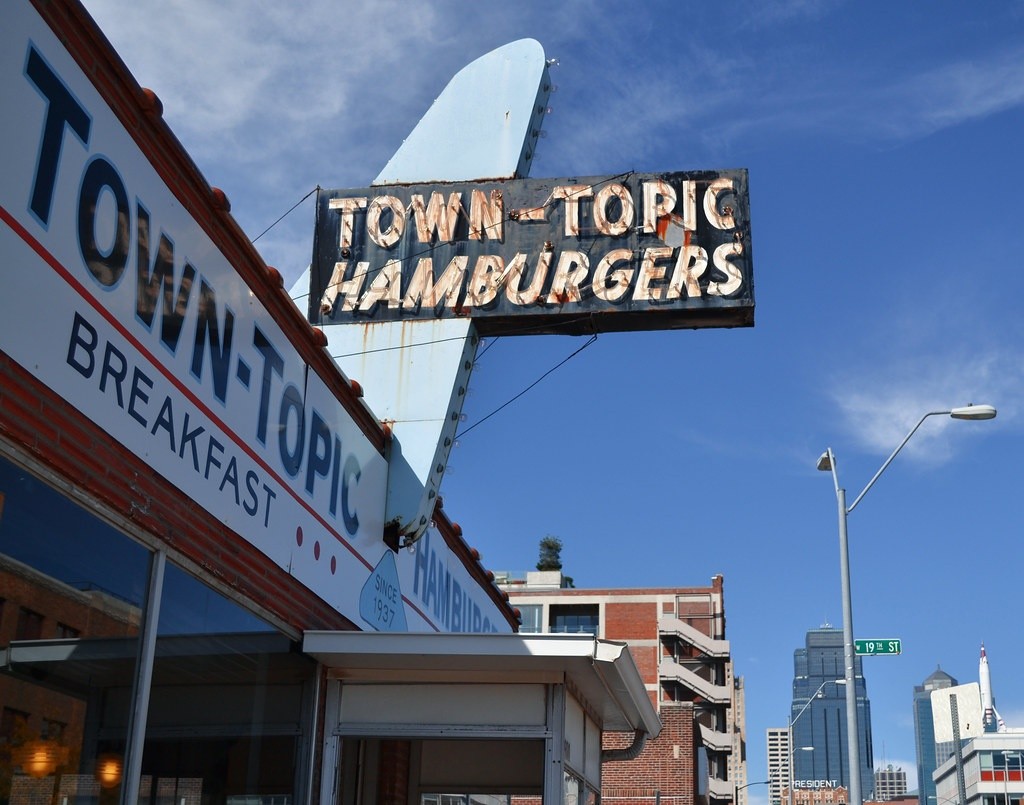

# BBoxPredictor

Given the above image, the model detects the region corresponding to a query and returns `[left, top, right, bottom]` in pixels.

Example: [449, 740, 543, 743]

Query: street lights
[769, 746, 814, 805]
[735, 781, 771, 805]
[815, 402, 996, 805]
[787, 679, 849, 805]
